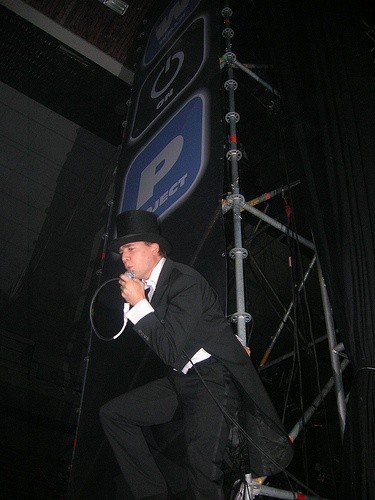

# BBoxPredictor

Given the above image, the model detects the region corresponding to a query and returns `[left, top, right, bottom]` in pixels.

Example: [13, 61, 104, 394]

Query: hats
[113, 211, 171, 254]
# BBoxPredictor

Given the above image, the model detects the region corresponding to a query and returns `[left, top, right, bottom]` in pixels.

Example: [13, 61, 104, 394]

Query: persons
[97, 210, 296, 500]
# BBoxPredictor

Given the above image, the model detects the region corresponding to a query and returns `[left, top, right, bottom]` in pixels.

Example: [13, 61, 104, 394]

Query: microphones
[122, 271, 135, 323]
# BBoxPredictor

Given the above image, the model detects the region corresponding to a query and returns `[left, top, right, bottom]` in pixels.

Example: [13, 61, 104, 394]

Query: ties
[143, 287, 150, 303]
